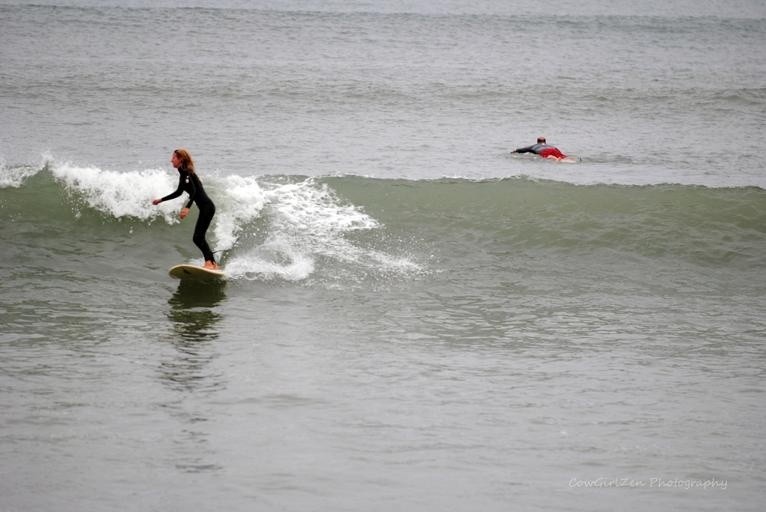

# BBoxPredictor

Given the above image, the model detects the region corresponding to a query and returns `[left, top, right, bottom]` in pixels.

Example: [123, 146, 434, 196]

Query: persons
[153, 148, 220, 271]
[511, 136, 578, 162]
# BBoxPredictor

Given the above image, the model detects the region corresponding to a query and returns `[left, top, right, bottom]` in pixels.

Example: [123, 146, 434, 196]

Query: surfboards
[170, 265, 226, 280]
